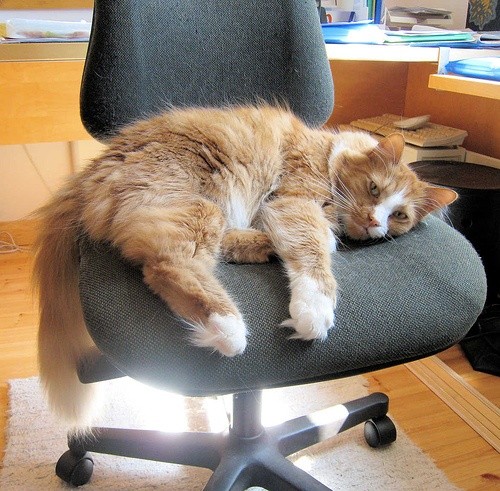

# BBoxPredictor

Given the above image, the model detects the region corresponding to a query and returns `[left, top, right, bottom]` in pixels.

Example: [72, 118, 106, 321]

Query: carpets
[0, 375, 458, 490]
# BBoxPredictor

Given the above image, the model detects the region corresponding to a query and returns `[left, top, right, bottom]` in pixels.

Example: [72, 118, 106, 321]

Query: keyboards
[349, 113, 468, 147]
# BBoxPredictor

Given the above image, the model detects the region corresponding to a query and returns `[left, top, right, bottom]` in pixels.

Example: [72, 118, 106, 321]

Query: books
[321, 7, 500, 81]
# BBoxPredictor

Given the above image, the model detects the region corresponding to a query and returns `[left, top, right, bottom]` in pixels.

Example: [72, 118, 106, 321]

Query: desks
[1, 27, 499, 159]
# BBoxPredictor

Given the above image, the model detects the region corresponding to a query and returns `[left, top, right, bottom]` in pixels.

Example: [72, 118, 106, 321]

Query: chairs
[54, 1, 488, 490]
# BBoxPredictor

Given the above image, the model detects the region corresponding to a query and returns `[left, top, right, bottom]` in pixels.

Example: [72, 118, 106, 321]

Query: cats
[22, 90, 459, 446]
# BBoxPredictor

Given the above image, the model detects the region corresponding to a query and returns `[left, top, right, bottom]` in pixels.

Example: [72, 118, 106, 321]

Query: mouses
[392, 114, 431, 131]
